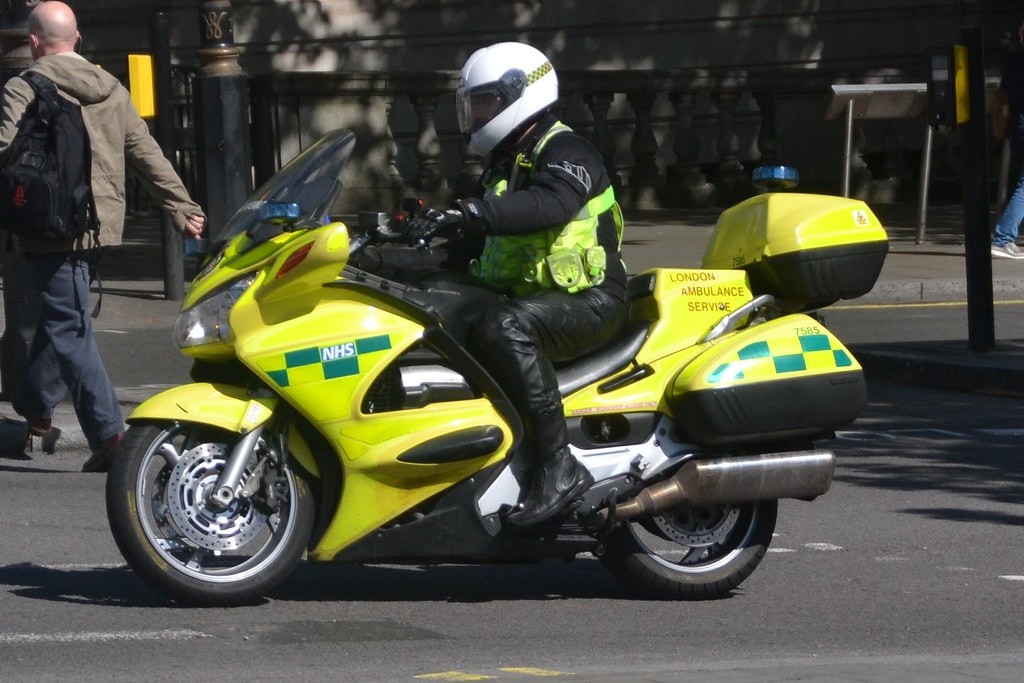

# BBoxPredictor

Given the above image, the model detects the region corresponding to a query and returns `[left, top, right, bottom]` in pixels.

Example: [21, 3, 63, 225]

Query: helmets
[455, 41, 560, 158]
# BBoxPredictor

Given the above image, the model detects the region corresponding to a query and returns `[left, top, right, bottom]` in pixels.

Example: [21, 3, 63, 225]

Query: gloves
[400, 197, 462, 251]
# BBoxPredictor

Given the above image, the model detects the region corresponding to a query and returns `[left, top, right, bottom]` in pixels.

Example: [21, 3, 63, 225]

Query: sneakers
[11, 394, 52, 437]
[80, 432, 125, 473]
[990, 242, 1024, 260]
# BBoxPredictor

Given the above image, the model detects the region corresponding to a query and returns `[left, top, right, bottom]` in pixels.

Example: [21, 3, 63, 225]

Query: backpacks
[0, 70, 105, 322]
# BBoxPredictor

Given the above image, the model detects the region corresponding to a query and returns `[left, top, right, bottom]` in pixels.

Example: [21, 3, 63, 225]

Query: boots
[506, 406, 596, 527]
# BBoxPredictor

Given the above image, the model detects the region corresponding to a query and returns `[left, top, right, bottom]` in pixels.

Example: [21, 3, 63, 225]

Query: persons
[0, 1, 207, 473]
[990, 19, 1023, 259]
[350, 41, 631, 526]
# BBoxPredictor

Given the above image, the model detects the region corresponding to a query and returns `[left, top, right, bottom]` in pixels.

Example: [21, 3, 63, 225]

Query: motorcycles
[107, 128, 890, 608]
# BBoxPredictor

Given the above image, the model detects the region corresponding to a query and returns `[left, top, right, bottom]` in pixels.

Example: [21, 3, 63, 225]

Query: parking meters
[924, 25, 995, 352]
